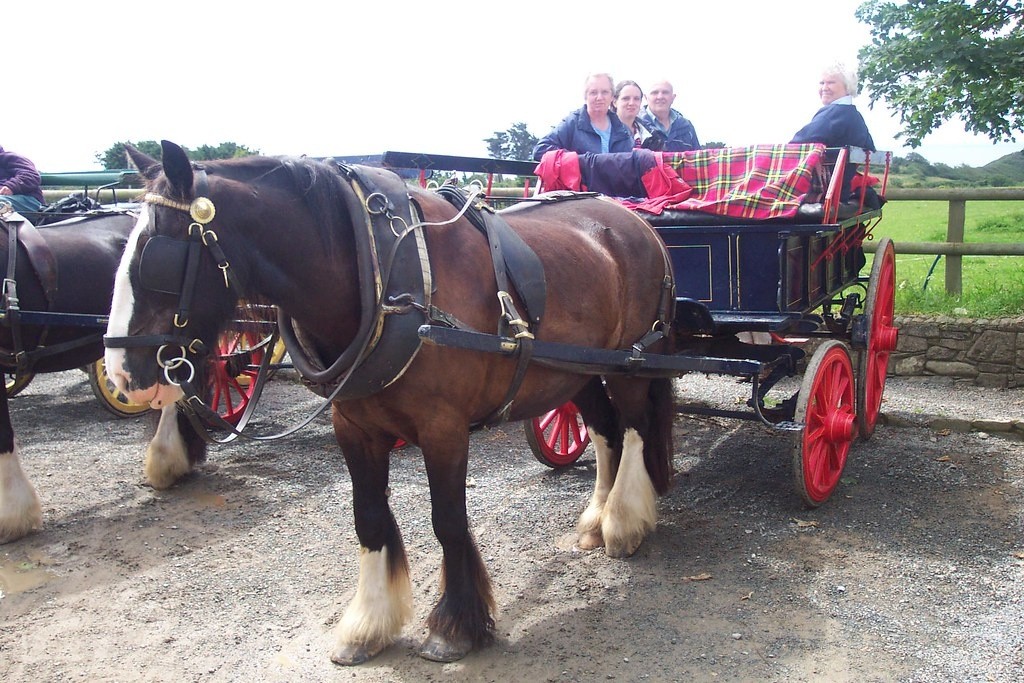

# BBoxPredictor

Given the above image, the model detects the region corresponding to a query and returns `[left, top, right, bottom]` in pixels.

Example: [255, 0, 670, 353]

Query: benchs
[775, 145, 893, 239]
[531, 143, 828, 236]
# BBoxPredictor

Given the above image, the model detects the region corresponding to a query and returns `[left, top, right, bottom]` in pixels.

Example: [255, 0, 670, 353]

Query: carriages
[0, 151, 545, 544]
[100, 138, 900, 666]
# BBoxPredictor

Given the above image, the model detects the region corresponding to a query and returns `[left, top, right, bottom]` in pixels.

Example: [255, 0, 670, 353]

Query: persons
[533, 74, 635, 162]
[639, 81, 702, 152]
[0, 146, 46, 222]
[612, 80, 667, 153]
[787, 64, 875, 202]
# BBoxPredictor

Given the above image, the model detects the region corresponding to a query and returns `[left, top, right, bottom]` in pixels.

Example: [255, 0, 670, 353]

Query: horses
[0, 140, 676, 666]
